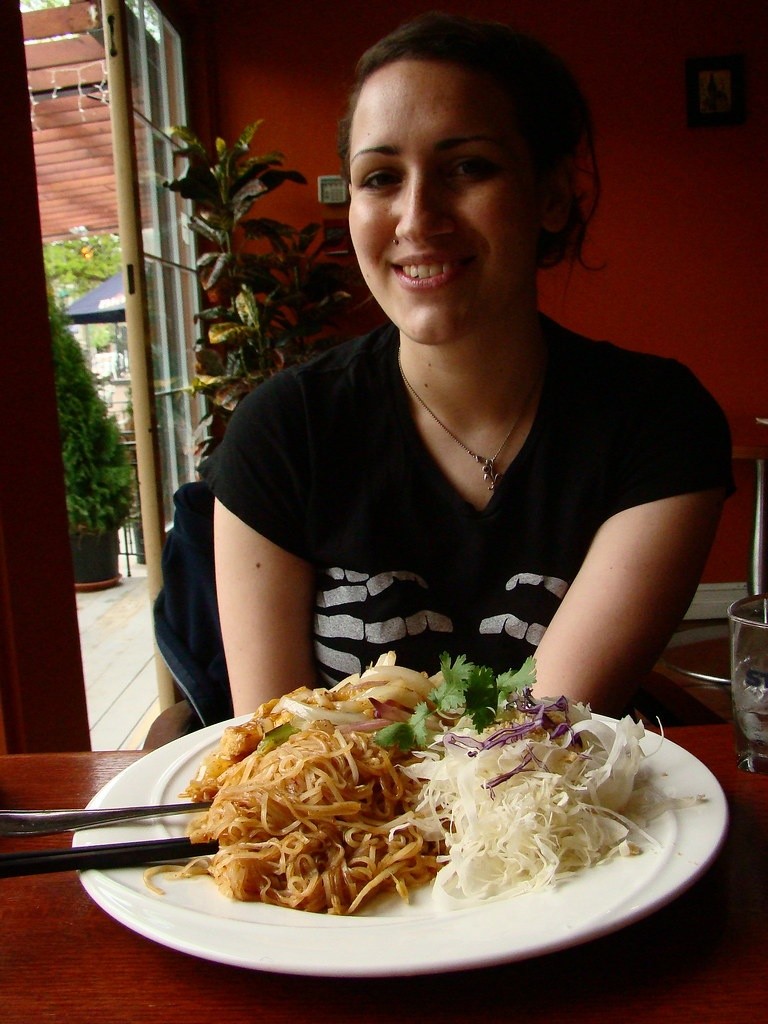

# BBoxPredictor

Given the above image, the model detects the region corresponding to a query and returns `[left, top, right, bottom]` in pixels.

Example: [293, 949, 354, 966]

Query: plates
[71, 713, 729, 978]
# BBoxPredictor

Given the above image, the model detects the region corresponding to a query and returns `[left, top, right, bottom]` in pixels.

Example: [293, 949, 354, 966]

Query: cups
[727, 595, 767, 775]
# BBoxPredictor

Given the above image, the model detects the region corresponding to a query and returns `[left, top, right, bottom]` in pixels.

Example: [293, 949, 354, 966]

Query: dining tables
[0, 720, 768, 1024]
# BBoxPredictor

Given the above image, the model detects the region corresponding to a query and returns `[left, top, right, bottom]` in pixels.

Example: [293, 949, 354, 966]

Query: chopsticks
[0, 837, 220, 879]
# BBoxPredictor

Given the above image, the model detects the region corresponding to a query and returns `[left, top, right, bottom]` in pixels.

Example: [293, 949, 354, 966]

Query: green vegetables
[373, 651, 537, 751]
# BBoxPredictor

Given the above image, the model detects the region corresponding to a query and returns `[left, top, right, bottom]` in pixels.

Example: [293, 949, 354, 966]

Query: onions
[269, 651, 453, 733]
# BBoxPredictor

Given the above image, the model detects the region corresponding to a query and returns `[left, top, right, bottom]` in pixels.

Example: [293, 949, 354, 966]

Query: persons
[155, 18, 735, 720]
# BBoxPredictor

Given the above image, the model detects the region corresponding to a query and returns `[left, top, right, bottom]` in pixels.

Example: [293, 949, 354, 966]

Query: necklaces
[401, 365, 534, 487]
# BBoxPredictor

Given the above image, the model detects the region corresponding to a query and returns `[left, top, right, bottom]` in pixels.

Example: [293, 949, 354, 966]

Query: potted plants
[46, 279, 135, 591]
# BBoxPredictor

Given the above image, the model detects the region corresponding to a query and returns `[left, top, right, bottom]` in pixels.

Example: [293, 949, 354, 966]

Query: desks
[661, 412, 768, 685]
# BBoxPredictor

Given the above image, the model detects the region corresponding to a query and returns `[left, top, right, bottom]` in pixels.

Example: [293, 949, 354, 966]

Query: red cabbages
[444, 681, 583, 800]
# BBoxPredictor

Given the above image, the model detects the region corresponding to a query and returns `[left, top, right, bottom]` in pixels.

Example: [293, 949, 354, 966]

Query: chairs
[144, 481, 728, 748]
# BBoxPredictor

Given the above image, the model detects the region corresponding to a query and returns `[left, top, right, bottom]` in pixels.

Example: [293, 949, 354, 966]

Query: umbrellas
[64, 270, 139, 324]
